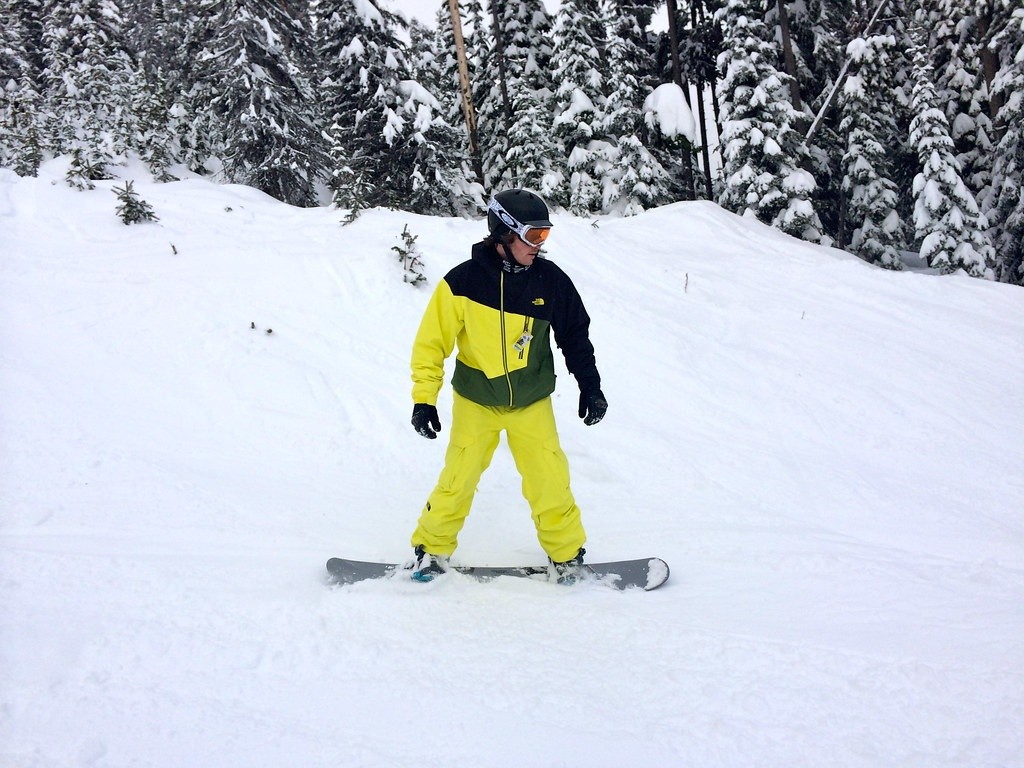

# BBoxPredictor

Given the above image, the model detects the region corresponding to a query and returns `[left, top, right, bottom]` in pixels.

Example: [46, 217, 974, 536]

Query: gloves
[411, 403, 441, 439]
[578, 387, 607, 425]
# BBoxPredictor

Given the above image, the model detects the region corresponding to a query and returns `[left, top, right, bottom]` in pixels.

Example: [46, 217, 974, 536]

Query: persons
[409, 188, 608, 585]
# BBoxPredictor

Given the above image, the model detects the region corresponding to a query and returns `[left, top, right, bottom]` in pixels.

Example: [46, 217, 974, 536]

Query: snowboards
[323, 556, 672, 591]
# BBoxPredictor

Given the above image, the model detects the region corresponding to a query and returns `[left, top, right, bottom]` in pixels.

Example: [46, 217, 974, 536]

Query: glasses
[521, 225, 551, 248]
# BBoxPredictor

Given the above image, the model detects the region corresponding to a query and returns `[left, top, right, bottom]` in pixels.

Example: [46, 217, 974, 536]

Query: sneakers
[549, 549, 586, 586]
[411, 546, 450, 583]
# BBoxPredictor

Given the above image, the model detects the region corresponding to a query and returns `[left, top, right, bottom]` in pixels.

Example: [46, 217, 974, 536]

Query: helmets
[487, 190, 553, 235]
